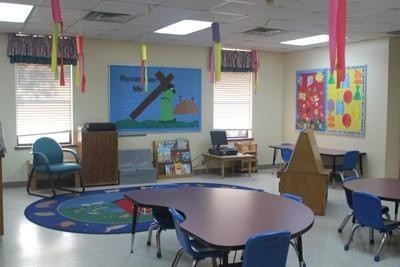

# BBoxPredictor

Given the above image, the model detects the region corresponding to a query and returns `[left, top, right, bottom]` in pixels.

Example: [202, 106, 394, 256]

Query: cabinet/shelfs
[153, 138, 193, 180]
[77, 127, 118, 187]
[235, 141, 258, 173]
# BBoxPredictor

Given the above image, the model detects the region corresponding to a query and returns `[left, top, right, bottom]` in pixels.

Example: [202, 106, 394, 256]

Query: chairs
[280, 142, 292, 168]
[281, 192, 307, 267]
[335, 150, 359, 183]
[343, 191, 400, 262]
[337, 177, 393, 236]
[219, 228, 291, 267]
[25, 136, 85, 201]
[146, 204, 175, 258]
[168, 207, 228, 266]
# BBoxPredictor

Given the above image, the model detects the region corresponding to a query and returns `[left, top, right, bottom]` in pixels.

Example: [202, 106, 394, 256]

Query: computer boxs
[209, 148, 237, 156]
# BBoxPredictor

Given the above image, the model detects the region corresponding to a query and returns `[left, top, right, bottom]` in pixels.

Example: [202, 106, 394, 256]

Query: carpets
[23, 182, 264, 234]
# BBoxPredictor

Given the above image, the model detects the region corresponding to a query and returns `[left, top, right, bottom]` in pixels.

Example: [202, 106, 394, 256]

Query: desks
[342, 177, 400, 220]
[122, 189, 315, 267]
[203, 153, 256, 179]
[25, 158, 81, 191]
[269, 145, 367, 189]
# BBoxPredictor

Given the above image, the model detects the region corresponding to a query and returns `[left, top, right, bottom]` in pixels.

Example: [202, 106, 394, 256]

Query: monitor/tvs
[210, 130, 228, 150]
